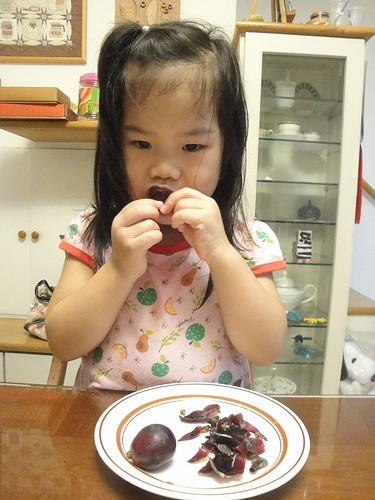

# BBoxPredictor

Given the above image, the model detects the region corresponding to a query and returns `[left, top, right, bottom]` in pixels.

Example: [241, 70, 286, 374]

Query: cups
[260, 127, 272, 136]
[273, 79, 295, 111]
[278, 123, 300, 134]
[302, 131, 321, 142]
[330, 4, 364, 27]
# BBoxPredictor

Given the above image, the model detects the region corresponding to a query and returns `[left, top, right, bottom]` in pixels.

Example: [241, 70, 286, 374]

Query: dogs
[339, 341, 375, 396]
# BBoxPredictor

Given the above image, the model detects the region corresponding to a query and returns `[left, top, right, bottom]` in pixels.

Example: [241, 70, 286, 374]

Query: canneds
[309, 10, 330, 25]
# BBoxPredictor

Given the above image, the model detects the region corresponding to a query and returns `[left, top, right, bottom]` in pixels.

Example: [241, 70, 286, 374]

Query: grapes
[148, 186, 172, 201]
[126, 423, 176, 471]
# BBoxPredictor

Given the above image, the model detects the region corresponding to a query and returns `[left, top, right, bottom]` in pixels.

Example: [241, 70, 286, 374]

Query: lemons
[248, 14, 265, 22]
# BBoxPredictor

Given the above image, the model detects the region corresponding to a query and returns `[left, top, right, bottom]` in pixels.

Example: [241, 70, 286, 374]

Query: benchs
[0, 317, 88, 386]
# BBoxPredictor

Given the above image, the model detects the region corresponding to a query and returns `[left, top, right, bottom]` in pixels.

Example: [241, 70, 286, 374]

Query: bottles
[77, 73, 100, 120]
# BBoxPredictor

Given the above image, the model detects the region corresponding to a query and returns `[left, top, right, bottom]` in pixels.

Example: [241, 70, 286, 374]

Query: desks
[0, 384, 375, 500]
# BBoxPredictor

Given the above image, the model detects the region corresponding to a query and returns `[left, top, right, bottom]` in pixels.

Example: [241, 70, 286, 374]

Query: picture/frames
[0, 0, 88, 65]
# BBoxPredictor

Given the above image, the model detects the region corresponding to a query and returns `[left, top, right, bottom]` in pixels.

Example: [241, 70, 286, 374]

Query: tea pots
[274, 269, 319, 313]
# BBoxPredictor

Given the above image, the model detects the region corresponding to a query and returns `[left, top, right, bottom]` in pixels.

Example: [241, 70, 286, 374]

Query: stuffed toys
[340, 342, 375, 395]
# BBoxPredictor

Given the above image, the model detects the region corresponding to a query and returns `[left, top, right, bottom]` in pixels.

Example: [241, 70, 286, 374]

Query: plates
[252, 376, 297, 395]
[273, 133, 303, 140]
[261, 78, 276, 113]
[93, 382, 310, 500]
[295, 82, 320, 117]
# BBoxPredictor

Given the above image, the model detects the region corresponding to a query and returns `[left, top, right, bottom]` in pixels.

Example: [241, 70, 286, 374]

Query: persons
[45, 20, 288, 392]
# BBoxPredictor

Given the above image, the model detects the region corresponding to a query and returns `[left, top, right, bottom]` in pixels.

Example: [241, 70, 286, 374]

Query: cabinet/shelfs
[229, 22, 375, 397]
